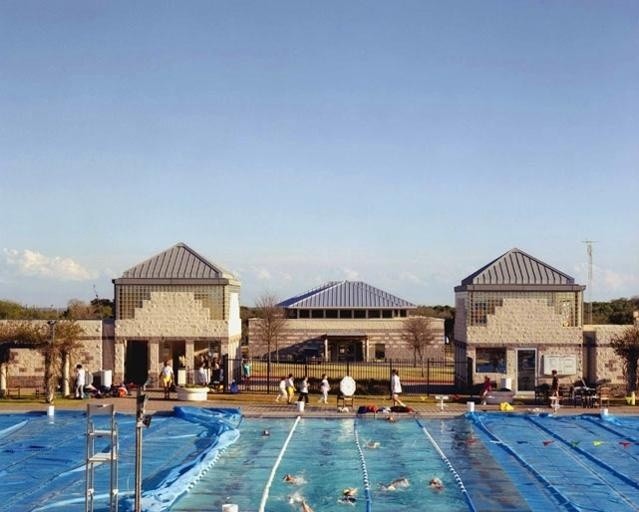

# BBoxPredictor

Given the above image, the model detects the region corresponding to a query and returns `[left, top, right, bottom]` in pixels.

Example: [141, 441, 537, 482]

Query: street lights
[46, 317, 59, 353]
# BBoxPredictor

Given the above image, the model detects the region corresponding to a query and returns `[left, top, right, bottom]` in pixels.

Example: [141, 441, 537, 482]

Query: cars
[292, 347, 324, 365]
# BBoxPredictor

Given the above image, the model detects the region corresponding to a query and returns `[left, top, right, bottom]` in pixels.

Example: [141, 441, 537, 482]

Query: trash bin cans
[101, 370, 112, 387]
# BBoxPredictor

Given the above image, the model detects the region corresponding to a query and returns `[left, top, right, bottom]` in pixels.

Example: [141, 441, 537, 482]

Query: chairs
[533, 384, 611, 409]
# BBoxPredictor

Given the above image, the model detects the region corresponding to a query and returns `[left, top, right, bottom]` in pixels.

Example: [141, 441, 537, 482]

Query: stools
[337, 396, 354, 410]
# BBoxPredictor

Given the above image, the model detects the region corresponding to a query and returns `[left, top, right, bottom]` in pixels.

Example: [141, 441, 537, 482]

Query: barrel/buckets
[222, 503, 239, 512]
[468, 402, 474, 412]
[601, 408, 608, 416]
[47, 406, 55, 416]
[295, 401, 305, 412]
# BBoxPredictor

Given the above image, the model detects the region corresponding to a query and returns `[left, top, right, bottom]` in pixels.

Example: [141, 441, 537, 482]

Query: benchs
[14, 385, 41, 399]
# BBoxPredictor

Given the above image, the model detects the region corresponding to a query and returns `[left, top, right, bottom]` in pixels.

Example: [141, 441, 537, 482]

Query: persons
[549, 369, 561, 408]
[158, 359, 175, 399]
[285, 373, 297, 404]
[479, 376, 492, 406]
[242, 360, 252, 392]
[73, 363, 86, 399]
[282, 473, 446, 511]
[70, 367, 79, 394]
[316, 373, 332, 404]
[390, 368, 406, 407]
[274, 375, 288, 404]
[197, 351, 224, 393]
[296, 375, 311, 404]
